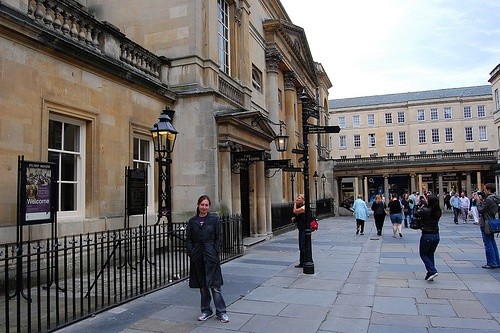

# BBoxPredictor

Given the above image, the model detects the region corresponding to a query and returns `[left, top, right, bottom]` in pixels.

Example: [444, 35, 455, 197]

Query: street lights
[320, 173, 327, 199]
[313, 171, 319, 200]
[290, 163, 295, 203]
[146, 114, 173, 226]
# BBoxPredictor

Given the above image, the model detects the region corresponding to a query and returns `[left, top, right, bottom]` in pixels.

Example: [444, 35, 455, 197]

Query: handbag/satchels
[410, 211, 422, 229]
[310, 220, 318, 231]
[488, 218, 500, 232]
[383, 202, 387, 216]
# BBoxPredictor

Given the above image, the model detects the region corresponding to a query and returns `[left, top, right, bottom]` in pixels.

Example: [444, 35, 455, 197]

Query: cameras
[477, 191, 484, 195]
[420, 196, 424, 199]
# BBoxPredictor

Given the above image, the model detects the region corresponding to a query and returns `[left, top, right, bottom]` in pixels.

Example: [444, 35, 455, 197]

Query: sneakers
[217, 313, 230, 323]
[198, 312, 212, 321]
[425, 272, 438, 281]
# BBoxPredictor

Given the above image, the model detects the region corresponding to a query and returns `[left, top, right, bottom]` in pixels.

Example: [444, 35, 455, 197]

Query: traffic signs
[306, 125, 341, 134]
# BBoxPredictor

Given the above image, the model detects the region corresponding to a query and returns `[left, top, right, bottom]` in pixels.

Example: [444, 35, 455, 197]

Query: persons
[27, 171, 51, 186]
[371, 195, 387, 236]
[186, 195, 230, 323]
[474, 183, 500, 269]
[444, 191, 462, 224]
[377, 190, 435, 228]
[352, 194, 370, 235]
[459, 192, 470, 224]
[469, 193, 480, 225]
[413, 195, 442, 281]
[292, 193, 314, 267]
[388, 193, 404, 238]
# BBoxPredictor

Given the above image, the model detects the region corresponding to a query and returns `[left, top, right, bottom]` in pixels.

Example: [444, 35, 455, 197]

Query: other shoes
[295, 265, 303, 268]
[494, 265, 500, 268]
[360, 233, 364, 235]
[482, 265, 495, 269]
[399, 233, 402, 237]
[356, 230, 359, 234]
[392, 235, 396, 238]
[474, 222, 479, 225]
[456, 222, 458, 224]
[377, 230, 381, 236]
[463, 220, 466, 224]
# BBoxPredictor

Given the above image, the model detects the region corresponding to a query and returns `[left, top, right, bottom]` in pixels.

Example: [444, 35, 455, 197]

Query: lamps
[251, 116, 289, 152]
